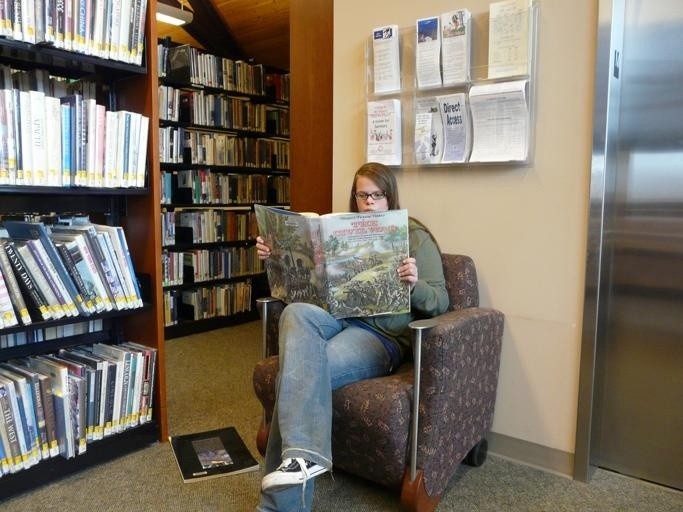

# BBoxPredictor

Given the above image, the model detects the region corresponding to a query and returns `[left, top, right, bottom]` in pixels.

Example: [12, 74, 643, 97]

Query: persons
[256, 162, 450, 511]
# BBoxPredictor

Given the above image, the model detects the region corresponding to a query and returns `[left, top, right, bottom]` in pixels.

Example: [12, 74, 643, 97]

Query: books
[254, 204, 411, 320]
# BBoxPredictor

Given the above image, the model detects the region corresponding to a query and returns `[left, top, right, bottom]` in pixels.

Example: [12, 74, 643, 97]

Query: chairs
[241, 250, 506, 510]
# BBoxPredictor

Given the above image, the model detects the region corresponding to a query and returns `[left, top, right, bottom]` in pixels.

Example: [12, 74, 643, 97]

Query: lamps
[152, 1, 194, 29]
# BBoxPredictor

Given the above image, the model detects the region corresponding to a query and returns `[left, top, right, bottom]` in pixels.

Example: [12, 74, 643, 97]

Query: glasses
[350, 189, 388, 201]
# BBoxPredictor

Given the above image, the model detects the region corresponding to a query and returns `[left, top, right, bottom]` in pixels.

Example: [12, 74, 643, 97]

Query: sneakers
[260, 455, 329, 496]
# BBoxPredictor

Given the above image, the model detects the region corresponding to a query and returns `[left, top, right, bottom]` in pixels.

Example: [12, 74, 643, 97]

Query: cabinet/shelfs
[0, 0, 169, 505]
[146, 32, 294, 340]
